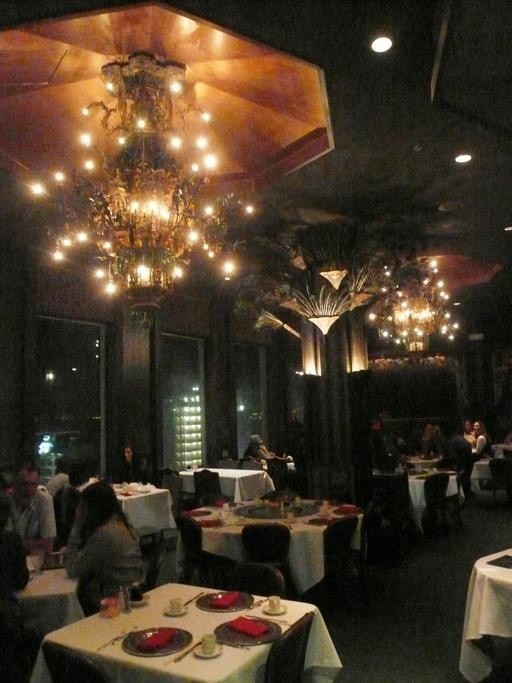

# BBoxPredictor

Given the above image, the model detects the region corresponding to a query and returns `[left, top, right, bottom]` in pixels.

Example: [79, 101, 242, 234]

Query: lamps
[0, 1, 335, 331]
[368, 259, 459, 345]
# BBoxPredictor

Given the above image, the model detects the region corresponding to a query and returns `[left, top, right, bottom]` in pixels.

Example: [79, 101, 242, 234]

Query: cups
[100, 597, 121, 619]
[171, 598, 182, 613]
[268, 596, 280, 611]
[202, 633, 215, 653]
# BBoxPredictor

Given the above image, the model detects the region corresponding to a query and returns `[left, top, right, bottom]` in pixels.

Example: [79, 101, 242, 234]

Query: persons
[64, 482, 144, 617]
[48, 456, 102, 555]
[364, 415, 492, 506]
[2, 496, 31, 635]
[241, 435, 276, 472]
[118, 442, 147, 485]
[1, 460, 57, 555]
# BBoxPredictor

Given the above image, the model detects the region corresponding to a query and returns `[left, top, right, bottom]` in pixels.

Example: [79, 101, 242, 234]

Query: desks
[14, 567, 84, 683]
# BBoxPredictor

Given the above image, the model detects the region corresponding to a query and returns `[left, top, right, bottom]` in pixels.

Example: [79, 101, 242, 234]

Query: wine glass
[23, 542, 47, 589]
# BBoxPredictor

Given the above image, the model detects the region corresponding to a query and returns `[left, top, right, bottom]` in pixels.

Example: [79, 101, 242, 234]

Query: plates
[264, 604, 286, 615]
[109, 480, 157, 497]
[196, 591, 253, 612]
[164, 607, 186, 616]
[193, 643, 223, 658]
[215, 617, 282, 647]
[123, 627, 193, 657]
[191, 498, 363, 533]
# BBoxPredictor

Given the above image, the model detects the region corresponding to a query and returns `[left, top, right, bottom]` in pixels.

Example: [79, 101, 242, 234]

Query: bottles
[120, 584, 131, 614]
[130, 581, 144, 606]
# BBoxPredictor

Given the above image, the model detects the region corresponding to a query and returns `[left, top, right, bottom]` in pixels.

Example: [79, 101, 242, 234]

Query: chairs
[41, 448, 511, 683]
